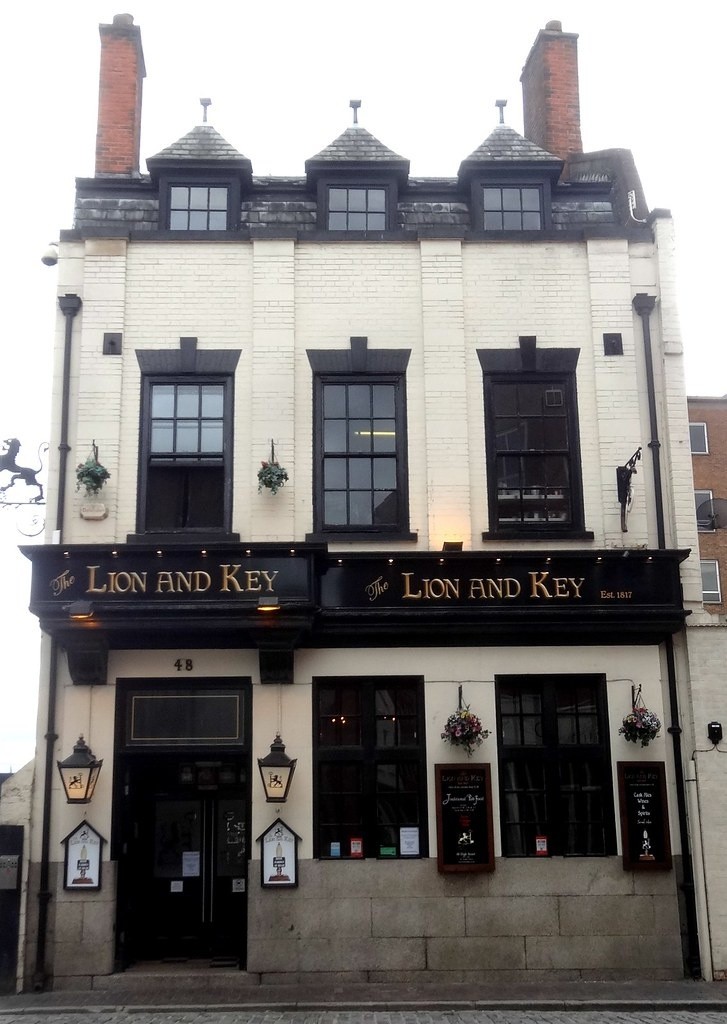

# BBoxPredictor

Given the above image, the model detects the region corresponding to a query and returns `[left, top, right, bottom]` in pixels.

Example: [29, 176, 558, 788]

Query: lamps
[257, 732, 298, 803]
[56, 733, 103, 804]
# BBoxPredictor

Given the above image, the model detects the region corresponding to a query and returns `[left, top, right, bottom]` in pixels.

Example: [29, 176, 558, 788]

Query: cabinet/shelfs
[498, 494, 568, 521]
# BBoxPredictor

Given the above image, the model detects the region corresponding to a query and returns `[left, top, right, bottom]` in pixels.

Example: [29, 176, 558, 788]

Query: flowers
[75, 458, 112, 497]
[257, 460, 289, 495]
[618, 706, 662, 748]
[441, 703, 492, 757]
